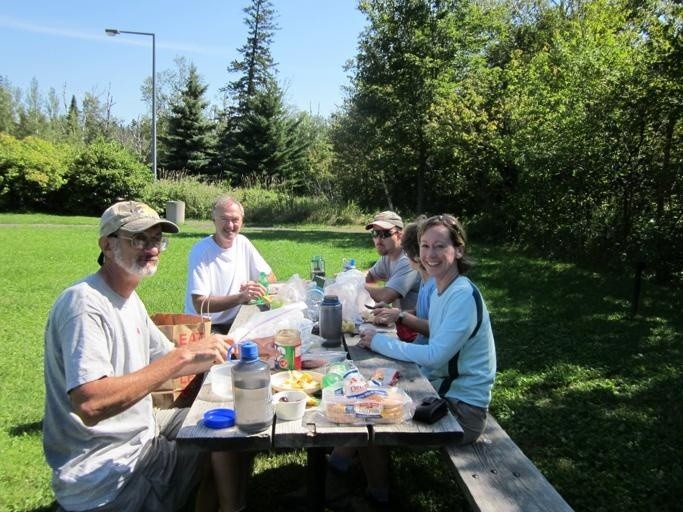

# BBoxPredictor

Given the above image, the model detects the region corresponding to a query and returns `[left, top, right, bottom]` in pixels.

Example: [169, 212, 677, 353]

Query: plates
[270, 372, 327, 393]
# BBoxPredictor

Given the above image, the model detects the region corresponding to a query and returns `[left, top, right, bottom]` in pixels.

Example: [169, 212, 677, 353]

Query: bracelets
[394, 310, 407, 324]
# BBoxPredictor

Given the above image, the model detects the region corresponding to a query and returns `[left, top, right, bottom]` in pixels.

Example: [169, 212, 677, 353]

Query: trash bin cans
[165, 200, 186, 225]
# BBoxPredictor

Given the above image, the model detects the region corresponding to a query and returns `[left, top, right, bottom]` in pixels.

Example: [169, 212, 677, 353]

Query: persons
[319, 213, 500, 512]
[360, 213, 438, 346]
[40, 200, 249, 511]
[330, 209, 425, 315]
[182, 195, 277, 337]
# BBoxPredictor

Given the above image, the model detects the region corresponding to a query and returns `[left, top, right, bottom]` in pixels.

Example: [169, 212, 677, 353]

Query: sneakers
[323, 487, 400, 511]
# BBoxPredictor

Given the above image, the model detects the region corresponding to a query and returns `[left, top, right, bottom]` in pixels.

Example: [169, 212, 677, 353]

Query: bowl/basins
[272, 390, 312, 421]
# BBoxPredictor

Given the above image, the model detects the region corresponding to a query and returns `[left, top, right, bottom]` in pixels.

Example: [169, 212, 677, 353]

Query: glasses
[368, 230, 398, 239]
[108, 234, 168, 250]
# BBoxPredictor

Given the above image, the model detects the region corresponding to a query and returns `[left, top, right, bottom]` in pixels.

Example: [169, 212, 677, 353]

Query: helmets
[99, 199, 180, 234]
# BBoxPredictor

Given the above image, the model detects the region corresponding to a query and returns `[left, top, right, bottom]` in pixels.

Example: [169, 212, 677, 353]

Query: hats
[365, 210, 403, 231]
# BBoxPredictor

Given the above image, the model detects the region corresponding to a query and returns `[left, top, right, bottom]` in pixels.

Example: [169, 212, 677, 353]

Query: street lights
[104, 28, 156, 183]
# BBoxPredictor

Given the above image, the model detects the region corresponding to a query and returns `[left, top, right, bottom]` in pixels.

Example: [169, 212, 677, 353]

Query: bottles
[253, 270, 272, 304]
[345, 260, 356, 270]
[273, 329, 304, 371]
[311, 256, 324, 281]
[228, 342, 275, 433]
[317, 294, 342, 345]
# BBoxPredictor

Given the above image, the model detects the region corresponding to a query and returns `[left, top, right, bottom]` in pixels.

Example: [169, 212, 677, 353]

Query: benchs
[443, 410, 575, 510]
